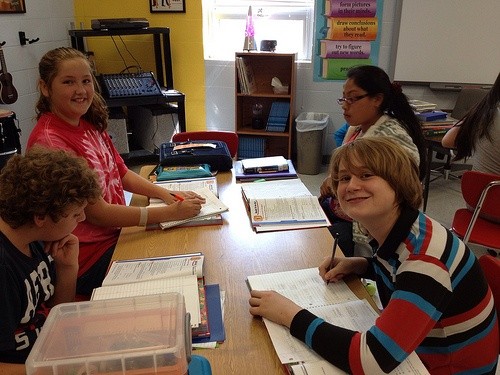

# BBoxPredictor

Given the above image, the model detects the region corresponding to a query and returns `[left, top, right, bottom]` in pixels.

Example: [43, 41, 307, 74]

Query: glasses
[336, 94, 370, 106]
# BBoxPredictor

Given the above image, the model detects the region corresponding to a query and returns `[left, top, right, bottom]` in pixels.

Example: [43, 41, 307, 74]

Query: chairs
[172, 132, 238, 158]
[448, 171, 500, 321]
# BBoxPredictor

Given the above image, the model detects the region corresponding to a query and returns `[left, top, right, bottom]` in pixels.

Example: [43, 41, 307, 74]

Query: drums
[0, 109, 21, 170]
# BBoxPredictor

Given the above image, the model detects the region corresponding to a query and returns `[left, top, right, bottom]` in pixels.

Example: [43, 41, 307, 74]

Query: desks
[423, 134, 455, 214]
[106, 160, 382, 375]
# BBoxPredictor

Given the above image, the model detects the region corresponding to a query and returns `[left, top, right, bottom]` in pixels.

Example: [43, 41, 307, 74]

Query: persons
[249, 137, 500, 375]
[441, 72, 500, 257]
[316, 65, 423, 255]
[0, 146, 100, 375]
[25, 47, 206, 299]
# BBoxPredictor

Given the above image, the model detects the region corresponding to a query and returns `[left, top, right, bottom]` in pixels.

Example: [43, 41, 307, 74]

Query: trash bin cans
[295, 112, 328, 175]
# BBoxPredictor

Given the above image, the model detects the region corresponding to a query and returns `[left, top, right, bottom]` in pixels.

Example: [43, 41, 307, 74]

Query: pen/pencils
[169, 192, 186, 200]
[327, 233, 341, 286]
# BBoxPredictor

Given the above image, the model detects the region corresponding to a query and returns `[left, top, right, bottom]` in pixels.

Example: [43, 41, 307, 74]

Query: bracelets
[138, 207, 148, 227]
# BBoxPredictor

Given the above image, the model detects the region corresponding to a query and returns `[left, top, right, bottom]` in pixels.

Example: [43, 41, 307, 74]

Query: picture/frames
[0, 0, 26, 13]
[149, 0, 186, 13]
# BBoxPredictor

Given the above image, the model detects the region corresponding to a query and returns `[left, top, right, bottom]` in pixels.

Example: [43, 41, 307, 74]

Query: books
[235, 57, 257, 95]
[145, 177, 230, 230]
[409, 99, 447, 122]
[246, 265, 431, 375]
[92, 252, 225, 349]
[235, 156, 332, 232]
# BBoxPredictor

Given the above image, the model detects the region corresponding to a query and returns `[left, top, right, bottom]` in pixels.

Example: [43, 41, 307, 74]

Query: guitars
[0, 46, 18, 105]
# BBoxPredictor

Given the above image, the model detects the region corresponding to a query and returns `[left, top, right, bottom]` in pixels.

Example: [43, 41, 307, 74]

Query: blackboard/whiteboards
[387, 0, 500, 90]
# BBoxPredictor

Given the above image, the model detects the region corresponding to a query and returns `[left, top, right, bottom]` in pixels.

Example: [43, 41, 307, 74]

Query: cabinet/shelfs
[235, 53, 295, 160]
[69, 26, 186, 134]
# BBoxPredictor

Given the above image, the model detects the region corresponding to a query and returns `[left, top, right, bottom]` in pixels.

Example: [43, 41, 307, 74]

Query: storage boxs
[25, 293, 192, 375]
